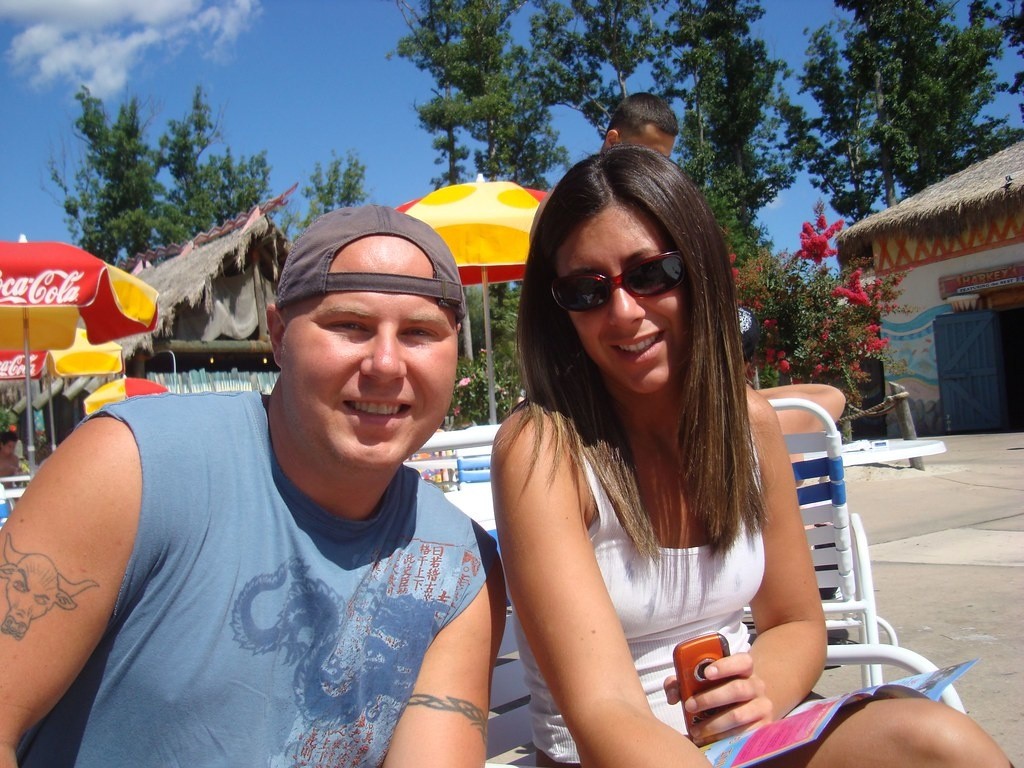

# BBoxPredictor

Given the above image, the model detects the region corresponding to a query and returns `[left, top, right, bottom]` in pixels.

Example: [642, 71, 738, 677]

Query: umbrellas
[0, 233, 170, 489]
[393, 172, 552, 426]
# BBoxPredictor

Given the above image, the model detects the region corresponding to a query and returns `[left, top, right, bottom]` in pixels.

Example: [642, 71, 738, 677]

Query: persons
[600, 93, 679, 158]
[0, 204, 508, 768]
[489, 147, 1020, 768]
[0, 431, 23, 488]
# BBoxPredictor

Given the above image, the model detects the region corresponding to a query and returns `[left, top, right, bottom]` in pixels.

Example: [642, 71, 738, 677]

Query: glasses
[551, 250, 686, 312]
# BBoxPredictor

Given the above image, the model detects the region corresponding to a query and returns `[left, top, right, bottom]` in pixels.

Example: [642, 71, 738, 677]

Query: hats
[275, 204, 466, 325]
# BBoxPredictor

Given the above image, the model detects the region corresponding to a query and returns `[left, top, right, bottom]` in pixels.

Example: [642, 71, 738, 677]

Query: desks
[841, 439, 946, 466]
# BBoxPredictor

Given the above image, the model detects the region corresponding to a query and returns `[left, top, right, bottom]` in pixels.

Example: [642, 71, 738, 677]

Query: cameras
[674, 631, 731, 738]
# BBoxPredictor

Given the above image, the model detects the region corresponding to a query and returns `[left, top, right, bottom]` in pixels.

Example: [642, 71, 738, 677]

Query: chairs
[445, 398, 964, 768]
